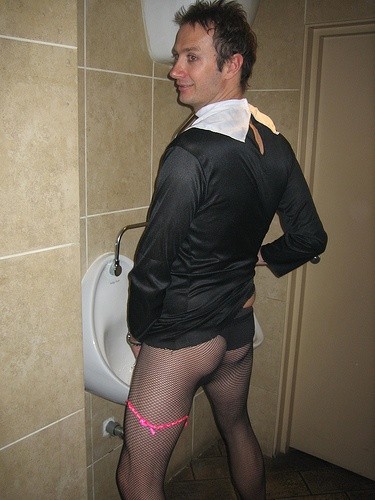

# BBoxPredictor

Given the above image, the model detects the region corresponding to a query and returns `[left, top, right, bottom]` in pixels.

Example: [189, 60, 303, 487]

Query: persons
[113, 0, 329, 500]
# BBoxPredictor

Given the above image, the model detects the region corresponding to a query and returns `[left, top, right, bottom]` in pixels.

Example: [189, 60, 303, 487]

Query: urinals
[81, 252, 140, 406]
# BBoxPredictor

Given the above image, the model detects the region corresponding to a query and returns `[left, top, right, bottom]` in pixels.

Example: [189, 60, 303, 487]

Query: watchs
[125, 331, 142, 348]
